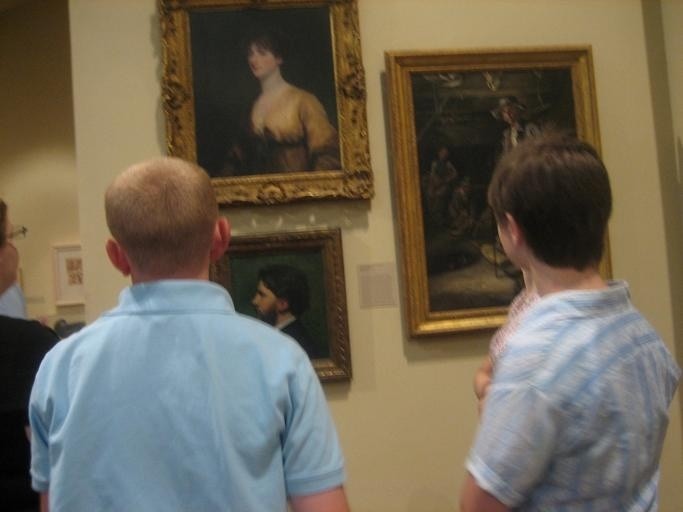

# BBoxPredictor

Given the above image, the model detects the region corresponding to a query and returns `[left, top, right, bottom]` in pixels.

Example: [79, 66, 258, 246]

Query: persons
[26, 155, 354, 512]
[457, 133, 682, 512]
[422, 92, 572, 292]
[245, 264, 318, 359]
[470, 266, 540, 420]
[217, 29, 340, 179]
[0, 196, 64, 511]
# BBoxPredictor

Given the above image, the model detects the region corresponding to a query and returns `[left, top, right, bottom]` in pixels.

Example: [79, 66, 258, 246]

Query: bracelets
[476, 379, 492, 402]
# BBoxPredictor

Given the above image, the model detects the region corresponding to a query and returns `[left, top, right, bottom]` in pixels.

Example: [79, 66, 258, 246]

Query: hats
[489, 96, 525, 121]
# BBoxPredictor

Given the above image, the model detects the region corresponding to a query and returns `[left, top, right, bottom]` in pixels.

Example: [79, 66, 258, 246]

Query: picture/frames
[384, 45, 613, 338]
[210, 227, 352, 383]
[158, 0, 374, 207]
[53, 243, 87, 309]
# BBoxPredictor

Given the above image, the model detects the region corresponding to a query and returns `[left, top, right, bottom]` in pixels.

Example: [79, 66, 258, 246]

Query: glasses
[4, 226, 27, 240]
